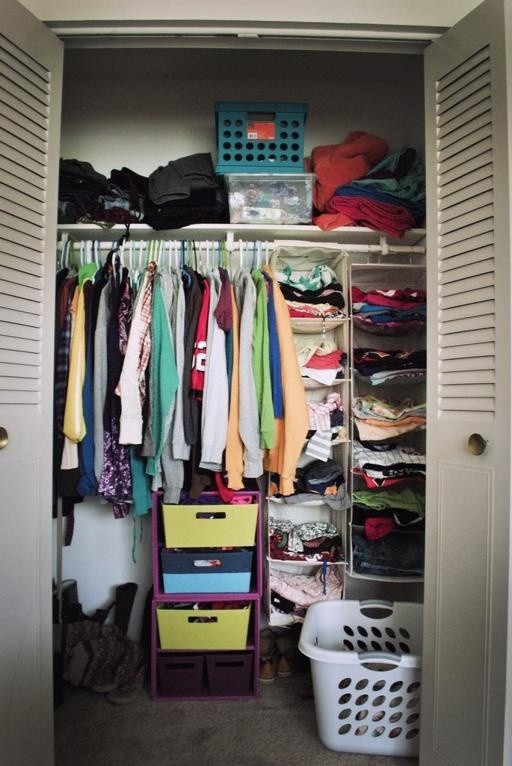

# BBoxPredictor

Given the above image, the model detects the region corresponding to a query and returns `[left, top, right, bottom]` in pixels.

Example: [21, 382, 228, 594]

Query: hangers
[55, 239, 269, 291]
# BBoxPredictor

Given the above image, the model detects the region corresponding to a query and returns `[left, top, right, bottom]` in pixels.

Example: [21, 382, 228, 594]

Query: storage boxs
[226, 173, 315, 227]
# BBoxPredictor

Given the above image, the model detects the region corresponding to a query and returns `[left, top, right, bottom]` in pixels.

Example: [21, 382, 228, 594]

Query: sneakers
[259, 627, 294, 683]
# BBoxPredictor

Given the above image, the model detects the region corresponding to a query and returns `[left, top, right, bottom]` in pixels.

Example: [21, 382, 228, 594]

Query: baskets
[299, 600, 424, 757]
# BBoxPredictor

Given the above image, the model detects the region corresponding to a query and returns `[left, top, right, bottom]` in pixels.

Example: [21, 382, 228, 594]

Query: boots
[53, 578, 145, 704]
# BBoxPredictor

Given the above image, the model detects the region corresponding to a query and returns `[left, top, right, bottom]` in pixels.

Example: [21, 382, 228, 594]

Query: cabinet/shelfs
[142, 484, 262, 702]
